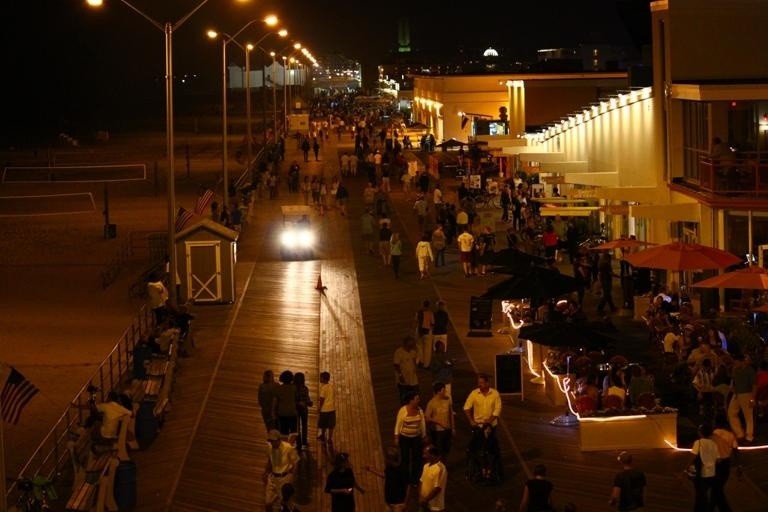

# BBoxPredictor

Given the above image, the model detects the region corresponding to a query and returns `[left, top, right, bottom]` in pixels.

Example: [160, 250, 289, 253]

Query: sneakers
[295, 435, 333, 450]
[417, 362, 430, 370]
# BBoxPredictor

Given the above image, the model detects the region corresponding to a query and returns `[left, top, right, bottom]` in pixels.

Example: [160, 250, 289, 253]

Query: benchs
[65, 305, 188, 512]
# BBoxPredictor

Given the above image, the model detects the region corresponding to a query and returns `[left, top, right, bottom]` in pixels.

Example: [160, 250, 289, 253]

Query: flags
[0, 366, 41, 425]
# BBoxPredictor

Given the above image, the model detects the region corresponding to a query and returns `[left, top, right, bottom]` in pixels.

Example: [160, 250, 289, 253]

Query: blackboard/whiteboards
[469, 295, 492, 329]
[495, 353, 523, 395]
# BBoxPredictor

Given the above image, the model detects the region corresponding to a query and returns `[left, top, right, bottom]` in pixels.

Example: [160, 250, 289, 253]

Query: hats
[263, 370, 274, 380]
[267, 428, 280, 441]
[337, 452, 350, 463]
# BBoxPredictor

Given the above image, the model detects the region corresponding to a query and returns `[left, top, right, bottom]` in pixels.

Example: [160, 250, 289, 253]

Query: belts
[271, 471, 289, 478]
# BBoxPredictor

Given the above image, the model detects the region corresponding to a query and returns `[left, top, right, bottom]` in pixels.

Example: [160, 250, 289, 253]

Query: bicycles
[474, 189, 503, 210]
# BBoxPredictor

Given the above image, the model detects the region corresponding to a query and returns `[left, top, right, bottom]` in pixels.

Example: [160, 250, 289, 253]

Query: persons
[360, 234, 767, 448]
[515, 461, 561, 511]
[682, 422, 720, 511]
[604, 450, 650, 510]
[708, 413, 744, 511]
[219, 85, 606, 234]
[69, 390, 135, 456]
[256, 367, 503, 511]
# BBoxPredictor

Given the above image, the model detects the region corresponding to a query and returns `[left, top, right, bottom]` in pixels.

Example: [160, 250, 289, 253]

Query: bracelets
[737, 464, 742, 468]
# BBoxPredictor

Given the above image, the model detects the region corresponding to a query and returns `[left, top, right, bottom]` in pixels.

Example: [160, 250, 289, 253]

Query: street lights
[82, 0, 250, 324]
[200, 6, 323, 223]
